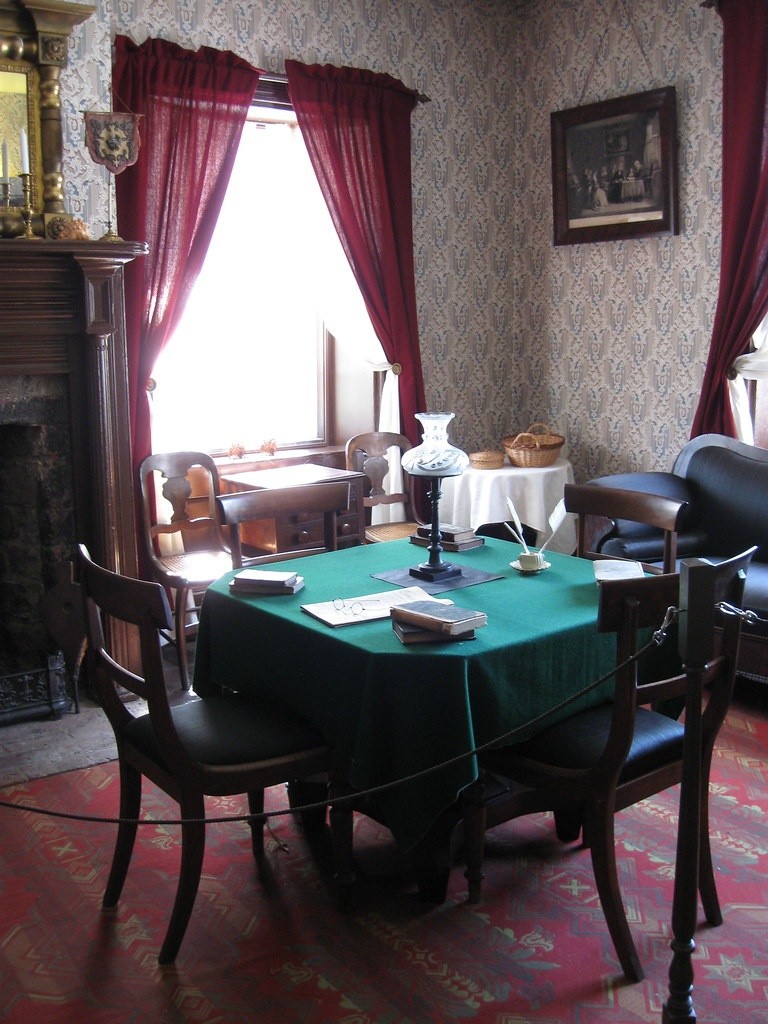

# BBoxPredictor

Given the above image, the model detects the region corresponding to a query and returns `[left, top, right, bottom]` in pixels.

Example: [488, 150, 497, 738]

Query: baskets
[469, 447, 505, 469]
[502, 423, 565, 468]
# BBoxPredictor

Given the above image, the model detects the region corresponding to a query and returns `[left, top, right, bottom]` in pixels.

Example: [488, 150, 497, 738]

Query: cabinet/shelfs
[221, 462, 366, 553]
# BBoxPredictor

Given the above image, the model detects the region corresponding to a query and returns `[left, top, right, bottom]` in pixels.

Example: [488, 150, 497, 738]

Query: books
[299, 584, 454, 629]
[229, 569, 306, 596]
[390, 600, 487, 644]
[408, 522, 485, 552]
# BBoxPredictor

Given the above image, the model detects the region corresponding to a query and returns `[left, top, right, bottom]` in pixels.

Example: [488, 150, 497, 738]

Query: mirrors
[0, 64, 40, 210]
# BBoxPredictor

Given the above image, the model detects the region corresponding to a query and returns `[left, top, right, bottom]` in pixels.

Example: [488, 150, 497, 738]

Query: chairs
[139, 451, 246, 690]
[345, 432, 424, 543]
[72, 543, 358, 964]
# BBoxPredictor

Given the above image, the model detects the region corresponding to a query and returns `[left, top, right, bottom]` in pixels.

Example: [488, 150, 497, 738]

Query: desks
[193, 536, 669, 909]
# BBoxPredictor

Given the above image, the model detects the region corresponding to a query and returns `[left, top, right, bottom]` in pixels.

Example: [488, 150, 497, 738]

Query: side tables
[440, 453, 578, 556]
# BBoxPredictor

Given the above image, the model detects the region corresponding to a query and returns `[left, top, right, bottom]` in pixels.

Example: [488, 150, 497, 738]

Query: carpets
[0, 689, 768, 1024]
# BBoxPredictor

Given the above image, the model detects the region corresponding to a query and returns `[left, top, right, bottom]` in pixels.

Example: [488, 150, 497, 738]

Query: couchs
[586, 435, 768, 686]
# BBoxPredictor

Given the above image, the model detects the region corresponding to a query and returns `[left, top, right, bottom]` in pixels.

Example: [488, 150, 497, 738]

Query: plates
[510, 561, 552, 574]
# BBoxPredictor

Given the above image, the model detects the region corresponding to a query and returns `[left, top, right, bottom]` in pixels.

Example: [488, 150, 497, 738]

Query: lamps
[500, 568, 744, 981]
[402, 412, 470, 583]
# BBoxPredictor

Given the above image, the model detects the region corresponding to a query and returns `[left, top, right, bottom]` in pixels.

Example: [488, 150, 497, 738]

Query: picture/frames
[549, 85, 680, 247]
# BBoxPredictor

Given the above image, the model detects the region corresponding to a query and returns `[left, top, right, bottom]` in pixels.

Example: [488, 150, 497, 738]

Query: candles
[19, 126, 29, 173]
[1, 139, 9, 182]
